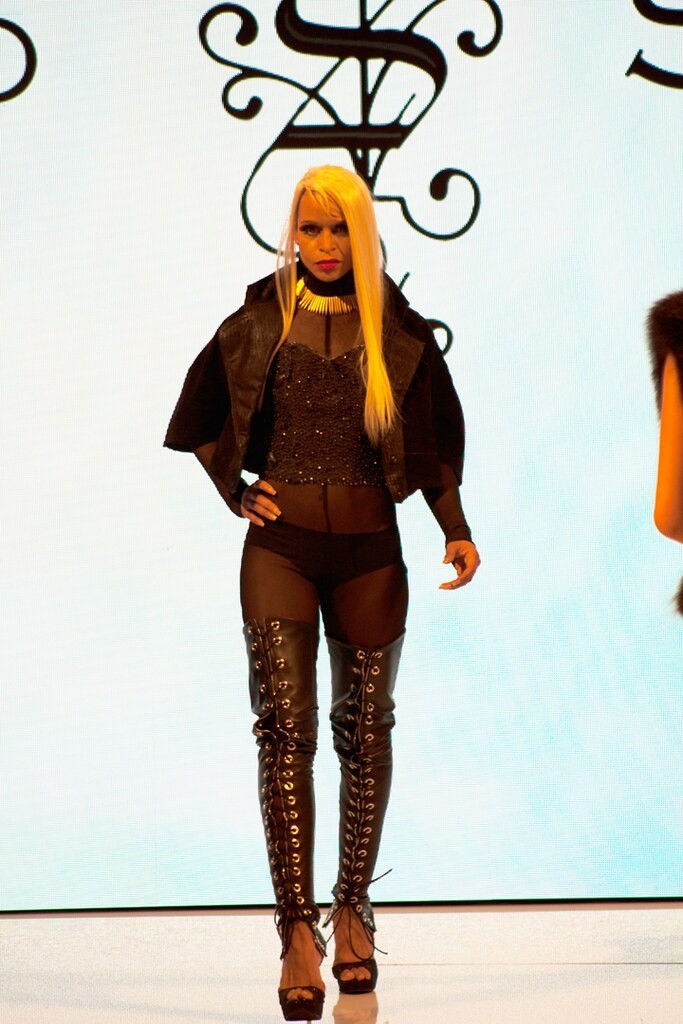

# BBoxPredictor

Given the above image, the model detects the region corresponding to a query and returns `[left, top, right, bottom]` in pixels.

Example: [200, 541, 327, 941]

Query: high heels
[332, 955, 377, 993]
[278, 928, 326, 1021]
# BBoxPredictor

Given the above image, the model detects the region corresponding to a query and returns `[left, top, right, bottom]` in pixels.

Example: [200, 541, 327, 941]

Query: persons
[644, 291, 683, 618]
[165, 165, 480, 1019]
